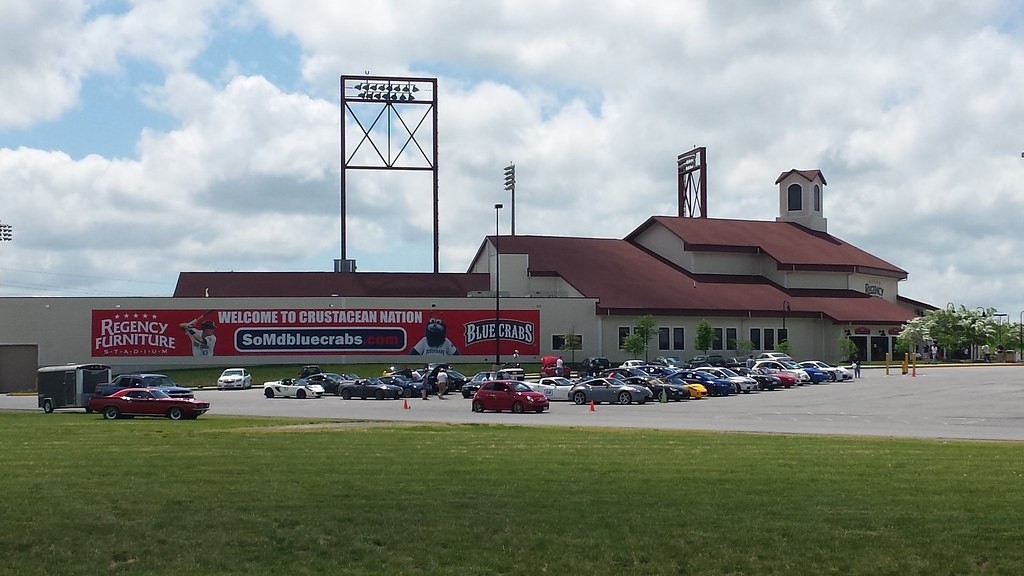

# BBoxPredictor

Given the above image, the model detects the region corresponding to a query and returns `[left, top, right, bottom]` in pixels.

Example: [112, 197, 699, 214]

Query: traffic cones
[403, 399, 408, 409]
[589, 400, 596, 412]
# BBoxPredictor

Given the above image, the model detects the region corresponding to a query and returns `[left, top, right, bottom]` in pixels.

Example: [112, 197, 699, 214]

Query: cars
[538, 356, 571, 379]
[568, 377, 653, 405]
[471, 381, 549, 414]
[594, 349, 856, 401]
[87, 388, 210, 421]
[340, 373, 359, 380]
[217, 368, 253, 391]
[461, 365, 512, 399]
[381, 362, 470, 400]
[303, 373, 346, 397]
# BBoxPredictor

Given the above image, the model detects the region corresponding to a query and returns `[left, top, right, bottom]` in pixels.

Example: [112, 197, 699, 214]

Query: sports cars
[338, 377, 403, 401]
[263, 377, 325, 400]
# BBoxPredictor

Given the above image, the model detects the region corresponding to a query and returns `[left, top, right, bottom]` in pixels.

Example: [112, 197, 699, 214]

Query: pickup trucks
[517, 375, 576, 401]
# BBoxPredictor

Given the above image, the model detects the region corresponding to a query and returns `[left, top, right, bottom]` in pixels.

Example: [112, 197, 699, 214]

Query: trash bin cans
[1005, 350, 1016, 362]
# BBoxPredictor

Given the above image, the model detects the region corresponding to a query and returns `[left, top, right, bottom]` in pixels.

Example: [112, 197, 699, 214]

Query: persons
[299, 368, 310, 378]
[481, 362, 520, 382]
[513, 350, 519, 361]
[981, 345, 992, 362]
[997, 343, 1004, 362]
[557, 356, 564, 376]
[853, 354, 861, 377]
[438, 369, 448, 398]
[401, 364, 428, 398]
[931, 345, 937, 363]
[746, 355, 755, 368]
[180, 320, 217, 356]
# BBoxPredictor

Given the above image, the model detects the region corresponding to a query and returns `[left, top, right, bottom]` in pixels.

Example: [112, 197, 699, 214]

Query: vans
[576, 356, 611, 379]
[297, 365, 324, 380]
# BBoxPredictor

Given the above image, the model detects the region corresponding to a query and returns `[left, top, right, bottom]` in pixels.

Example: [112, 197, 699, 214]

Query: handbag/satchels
[852, 363, 857, 368]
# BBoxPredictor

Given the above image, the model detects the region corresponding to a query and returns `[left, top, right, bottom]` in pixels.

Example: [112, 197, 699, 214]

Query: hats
[202, 321, 218, 329]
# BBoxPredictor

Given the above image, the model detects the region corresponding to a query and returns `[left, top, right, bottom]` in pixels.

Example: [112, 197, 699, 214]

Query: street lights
[781, 300, 792, 338]
[503, 163, 518, 235]
[492, 203, 506, 373]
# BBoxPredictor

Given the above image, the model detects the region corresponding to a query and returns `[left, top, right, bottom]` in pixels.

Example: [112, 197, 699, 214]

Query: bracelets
[189, 329, 195, 335]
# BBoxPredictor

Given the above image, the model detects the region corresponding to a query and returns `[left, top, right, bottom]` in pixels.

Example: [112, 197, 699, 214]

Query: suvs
[95, 372, 195, 400]
